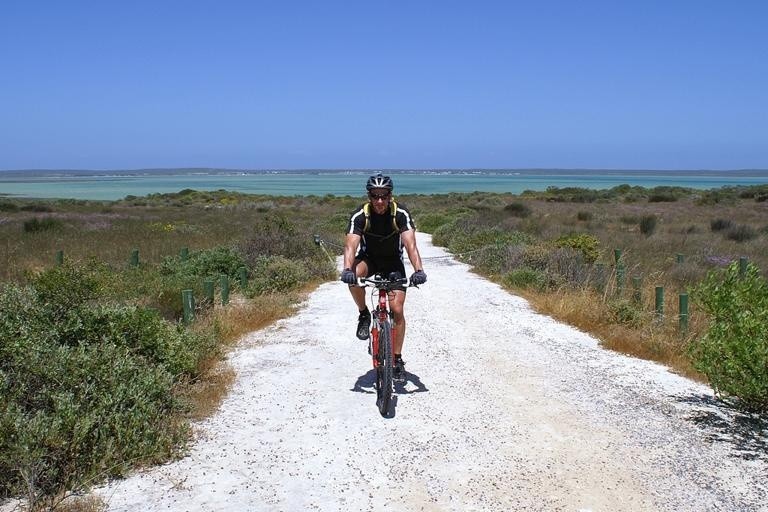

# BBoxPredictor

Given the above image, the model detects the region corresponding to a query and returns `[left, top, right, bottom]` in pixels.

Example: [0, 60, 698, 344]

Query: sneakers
[356, 306, 371, 340]
[392, 353, 405, 383]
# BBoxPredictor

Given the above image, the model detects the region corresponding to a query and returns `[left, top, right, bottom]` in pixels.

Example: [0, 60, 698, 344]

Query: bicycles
[345, 270, 427, 417]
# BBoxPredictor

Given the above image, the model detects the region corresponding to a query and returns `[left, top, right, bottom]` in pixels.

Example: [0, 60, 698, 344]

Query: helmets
[366, 174, 393, 191]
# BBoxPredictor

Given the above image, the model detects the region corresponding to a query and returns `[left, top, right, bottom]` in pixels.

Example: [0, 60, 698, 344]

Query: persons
[341, 174, 427, 381]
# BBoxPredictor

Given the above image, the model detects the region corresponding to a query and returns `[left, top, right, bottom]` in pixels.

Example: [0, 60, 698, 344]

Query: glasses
[371, 192, 387, 200]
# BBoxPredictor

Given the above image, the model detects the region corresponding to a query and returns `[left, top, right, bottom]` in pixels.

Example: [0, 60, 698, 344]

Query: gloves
[339, 268, 358, 284]
[410, 271, 427, 284]
